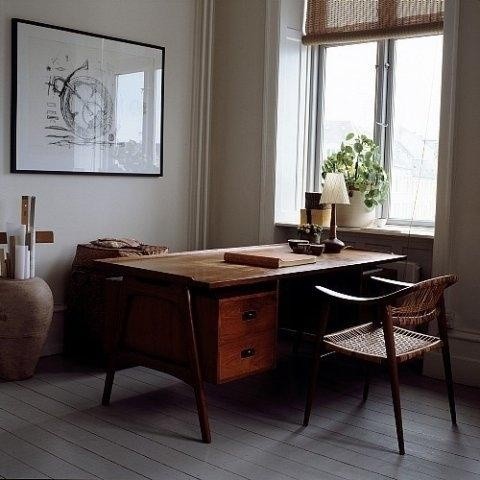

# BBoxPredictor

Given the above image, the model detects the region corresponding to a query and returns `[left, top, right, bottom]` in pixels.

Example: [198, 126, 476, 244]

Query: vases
[300, 233, 321, 244]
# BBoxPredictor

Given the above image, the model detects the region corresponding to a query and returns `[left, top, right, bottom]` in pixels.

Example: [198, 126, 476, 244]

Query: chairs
[302, 271, 463, 454]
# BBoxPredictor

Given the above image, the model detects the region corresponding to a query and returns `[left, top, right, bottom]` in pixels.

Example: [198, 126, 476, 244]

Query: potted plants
[322, 133, 391, 228]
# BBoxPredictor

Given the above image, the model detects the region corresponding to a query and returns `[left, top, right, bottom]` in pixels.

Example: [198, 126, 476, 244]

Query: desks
[90, 242, 408, 444]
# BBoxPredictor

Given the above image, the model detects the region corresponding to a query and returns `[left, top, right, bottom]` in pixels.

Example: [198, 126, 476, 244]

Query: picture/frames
[10, 17, 165, 177]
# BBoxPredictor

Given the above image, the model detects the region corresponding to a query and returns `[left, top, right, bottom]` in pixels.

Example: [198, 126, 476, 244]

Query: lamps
[319, 172, 351, 253]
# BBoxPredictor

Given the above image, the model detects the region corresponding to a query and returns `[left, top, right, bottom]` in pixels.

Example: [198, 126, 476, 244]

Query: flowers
[297, 222, 323, 234]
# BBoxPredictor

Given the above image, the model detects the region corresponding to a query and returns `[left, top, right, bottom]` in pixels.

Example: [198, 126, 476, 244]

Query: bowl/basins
[288, 239, 325, 257]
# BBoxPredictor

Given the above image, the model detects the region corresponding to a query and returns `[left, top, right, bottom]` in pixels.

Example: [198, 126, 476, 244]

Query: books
[223, 251, 317, 268]
